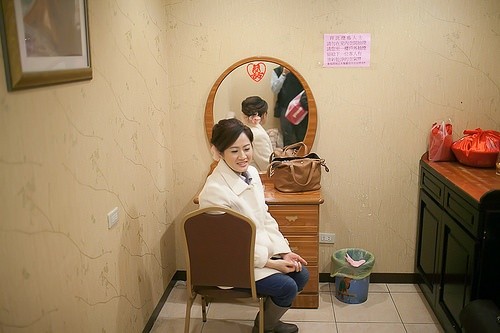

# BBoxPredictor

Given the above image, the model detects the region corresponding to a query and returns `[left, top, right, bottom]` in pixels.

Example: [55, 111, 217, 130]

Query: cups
[496, 152, 500, 175]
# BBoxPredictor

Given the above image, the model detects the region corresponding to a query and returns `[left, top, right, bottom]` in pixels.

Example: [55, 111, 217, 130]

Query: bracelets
[282, 74, 285, 76]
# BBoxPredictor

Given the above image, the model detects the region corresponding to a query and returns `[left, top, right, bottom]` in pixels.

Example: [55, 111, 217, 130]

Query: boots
[252, 296, 298, 333]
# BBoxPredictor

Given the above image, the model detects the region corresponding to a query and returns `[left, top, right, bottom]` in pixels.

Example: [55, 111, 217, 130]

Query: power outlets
[107, 208, 119, 229]
[320, 233, 336, 243]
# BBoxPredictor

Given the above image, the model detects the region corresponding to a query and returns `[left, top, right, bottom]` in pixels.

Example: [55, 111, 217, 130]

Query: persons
[237, 96, 273, 172]
[271, 65, 308, 153]
[198, 119, 310, 333]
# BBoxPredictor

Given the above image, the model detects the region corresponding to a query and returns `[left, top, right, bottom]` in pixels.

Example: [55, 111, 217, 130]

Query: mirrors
[205, 56, 317, 181]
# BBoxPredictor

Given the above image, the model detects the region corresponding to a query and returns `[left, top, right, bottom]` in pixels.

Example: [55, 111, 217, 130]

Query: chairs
[181, 206, 265, 333]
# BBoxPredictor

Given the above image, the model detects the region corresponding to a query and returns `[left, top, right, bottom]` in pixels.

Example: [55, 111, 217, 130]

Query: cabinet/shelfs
[415, 151, 500, 333]
[193, 180, 324, 310]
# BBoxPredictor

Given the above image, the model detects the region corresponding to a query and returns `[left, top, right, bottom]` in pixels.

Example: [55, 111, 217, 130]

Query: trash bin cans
[330, 247, 376, 304]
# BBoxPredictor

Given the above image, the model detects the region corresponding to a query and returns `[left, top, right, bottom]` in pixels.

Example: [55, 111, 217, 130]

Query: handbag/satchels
[266, 152, 329, 192]
[270, 142, 307, 164]
[285, 90, 308, 125]
[428, 120, 500, 167]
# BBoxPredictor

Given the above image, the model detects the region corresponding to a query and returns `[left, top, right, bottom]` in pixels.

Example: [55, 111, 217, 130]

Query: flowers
[244, 114, 261, 125]
[211, 144, 221, 163]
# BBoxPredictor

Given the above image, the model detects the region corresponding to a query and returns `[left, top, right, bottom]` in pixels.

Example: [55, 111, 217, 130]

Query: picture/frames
[0, 0, 93, 93]
